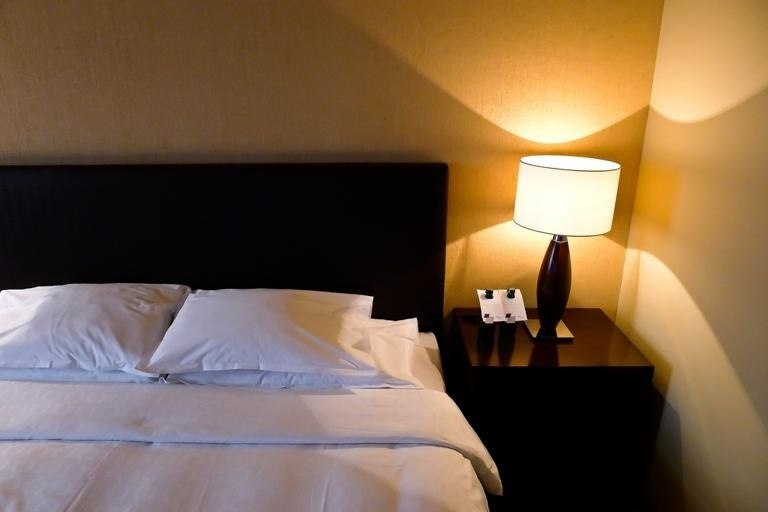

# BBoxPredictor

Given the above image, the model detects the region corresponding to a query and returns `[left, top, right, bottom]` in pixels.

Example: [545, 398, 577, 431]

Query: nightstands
[447, 307, 654, 495]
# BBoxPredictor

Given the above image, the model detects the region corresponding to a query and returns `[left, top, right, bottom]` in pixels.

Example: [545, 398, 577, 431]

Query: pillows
[0, 283, 187, 378]
[140, 288, 374, 380]
[167, 312, 418, 389]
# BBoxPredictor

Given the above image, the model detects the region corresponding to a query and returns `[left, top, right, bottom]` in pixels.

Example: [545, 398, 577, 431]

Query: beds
[1, 161, 503, 512]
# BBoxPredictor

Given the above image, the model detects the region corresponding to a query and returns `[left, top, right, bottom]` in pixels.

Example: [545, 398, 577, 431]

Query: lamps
[513, 155, 623, 339]
